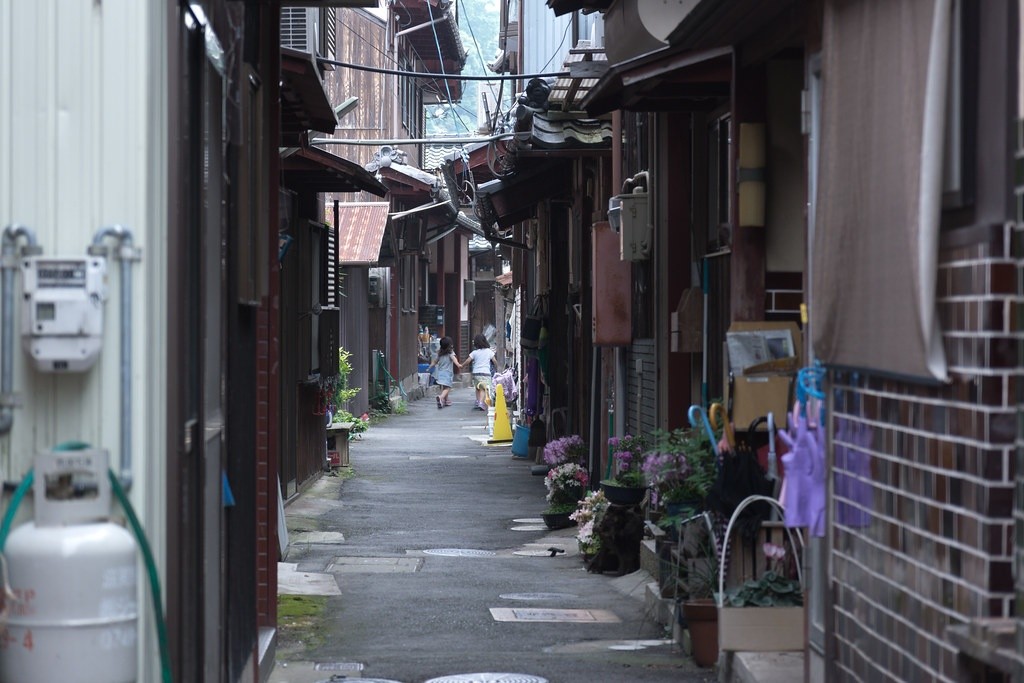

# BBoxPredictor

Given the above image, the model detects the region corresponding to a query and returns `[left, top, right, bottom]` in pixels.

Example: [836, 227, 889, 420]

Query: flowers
[542, 434, 589, 507]
[568, 489, 612, 552]
[606, 433, 647, 486]
[733, 541, 805, 608]
[639, 428, 711, 530]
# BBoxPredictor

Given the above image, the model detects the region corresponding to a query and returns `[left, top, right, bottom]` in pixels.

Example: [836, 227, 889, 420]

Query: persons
[426, 336, 460, 408]
[460, 334, 497, 411]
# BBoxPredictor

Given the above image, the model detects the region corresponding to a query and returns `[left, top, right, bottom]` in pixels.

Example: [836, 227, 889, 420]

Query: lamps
[736, 124, 769, 227]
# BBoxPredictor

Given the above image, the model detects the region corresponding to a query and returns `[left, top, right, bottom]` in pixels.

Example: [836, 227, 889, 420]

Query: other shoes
[436, 395, 443, 407]
[444, 398, 453, 405]
[475, 399, 478, 405]
[478, 401, 486, 411]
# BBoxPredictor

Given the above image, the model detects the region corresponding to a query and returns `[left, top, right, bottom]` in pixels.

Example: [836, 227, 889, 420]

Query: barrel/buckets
[511, 424, 531, 457]
[487, 407, 511, 435]
[417, 363, 434, 386]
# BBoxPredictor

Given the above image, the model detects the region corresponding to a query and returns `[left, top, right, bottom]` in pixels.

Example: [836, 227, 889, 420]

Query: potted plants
[624, 516, 719, 669]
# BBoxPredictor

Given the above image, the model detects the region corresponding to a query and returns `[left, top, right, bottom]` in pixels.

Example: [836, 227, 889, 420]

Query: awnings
[282, 144, 389, 198]
[325, 201, 388, 266]
[579, 46, 733, 119]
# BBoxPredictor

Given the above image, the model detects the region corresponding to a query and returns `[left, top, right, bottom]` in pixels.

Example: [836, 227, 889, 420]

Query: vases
[600, 479, 650, 507]
[714, 497, 806, 652]
[539, 508, 577, 530]
[650, 509, 684, 555]
[573, 543, 596, 562]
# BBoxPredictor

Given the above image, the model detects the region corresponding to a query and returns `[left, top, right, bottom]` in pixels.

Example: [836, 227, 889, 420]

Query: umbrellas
[689, 403, 774, 550]
